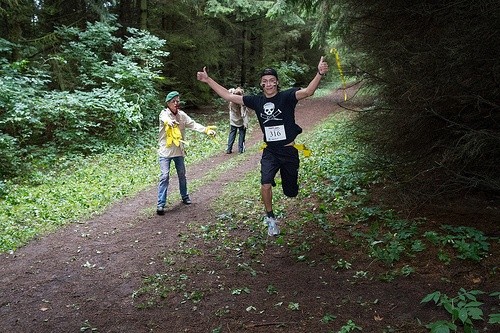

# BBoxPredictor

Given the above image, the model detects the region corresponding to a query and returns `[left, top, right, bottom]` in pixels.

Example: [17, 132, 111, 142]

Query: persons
[157, 91, 216, 215]
[227, 87, 246, 153]
[197, 55, 328, 237]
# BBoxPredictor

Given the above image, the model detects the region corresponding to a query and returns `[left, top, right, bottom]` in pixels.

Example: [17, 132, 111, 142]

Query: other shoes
[157, 206, 164, 215]
[183, 197, 191, 204]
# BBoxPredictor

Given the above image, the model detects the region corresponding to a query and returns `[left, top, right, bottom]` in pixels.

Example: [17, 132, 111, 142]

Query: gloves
[168, 120, 180, 127]
[208, 129, 216, 135]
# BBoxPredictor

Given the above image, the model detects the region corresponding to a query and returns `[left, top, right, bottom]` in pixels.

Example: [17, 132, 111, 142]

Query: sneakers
[264, 217, 280, 237]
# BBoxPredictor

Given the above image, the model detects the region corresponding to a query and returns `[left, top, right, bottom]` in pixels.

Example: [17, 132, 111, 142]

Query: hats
[261, 67, 278, 80]
[165, 91, 180, 103]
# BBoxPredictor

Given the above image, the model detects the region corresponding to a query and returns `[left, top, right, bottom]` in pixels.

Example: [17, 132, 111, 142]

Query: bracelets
[319, 72, 327, 76]
